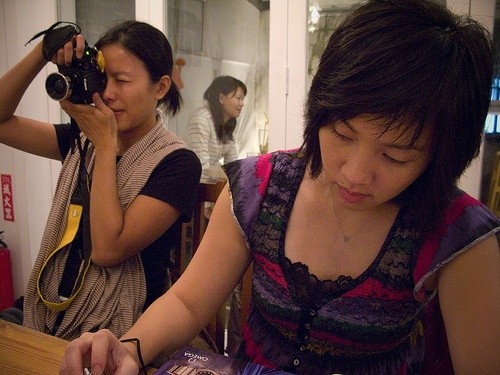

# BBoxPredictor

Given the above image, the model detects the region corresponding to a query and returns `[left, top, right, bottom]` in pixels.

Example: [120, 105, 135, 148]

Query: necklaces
[330, 188, 382, 243]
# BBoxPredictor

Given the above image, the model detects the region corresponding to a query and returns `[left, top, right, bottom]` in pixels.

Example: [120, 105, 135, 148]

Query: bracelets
[119, 338, 148, 375]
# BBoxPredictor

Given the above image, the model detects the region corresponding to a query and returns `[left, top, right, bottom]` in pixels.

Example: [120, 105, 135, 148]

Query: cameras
[45, 48, 107, 106]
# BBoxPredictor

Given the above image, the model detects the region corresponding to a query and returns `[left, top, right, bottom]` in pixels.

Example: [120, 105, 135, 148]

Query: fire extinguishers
[0, 231, 15, 313]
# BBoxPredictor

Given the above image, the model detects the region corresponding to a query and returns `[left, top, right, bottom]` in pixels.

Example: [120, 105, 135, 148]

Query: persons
[0, 20, 202, 342]
[59, 0, 500, 375]
[188, 76, 247, 183]
